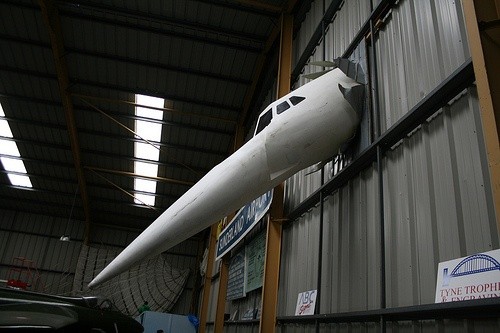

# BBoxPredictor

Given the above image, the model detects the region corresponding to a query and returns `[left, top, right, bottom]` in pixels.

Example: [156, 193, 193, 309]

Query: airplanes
[87, 68, 360, 288]
